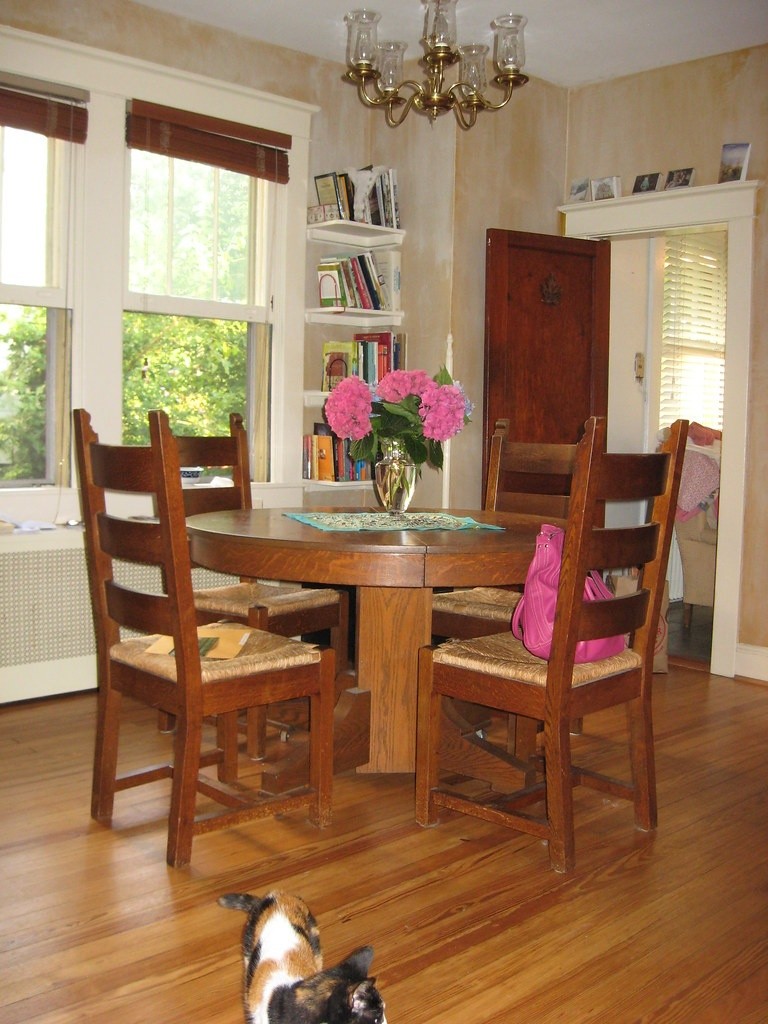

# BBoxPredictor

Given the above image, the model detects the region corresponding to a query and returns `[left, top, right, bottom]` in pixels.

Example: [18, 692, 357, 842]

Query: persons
[666, 174, 682, 188]
[640, 178, 649, 190]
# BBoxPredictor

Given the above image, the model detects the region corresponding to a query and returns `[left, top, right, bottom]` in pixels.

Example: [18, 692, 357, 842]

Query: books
[718, 143, 751, 183]
[318, 250, 401, 312]
[314, 163, 401, 229]
[321, 331, 408, 392]
[303, 421, 384, 482]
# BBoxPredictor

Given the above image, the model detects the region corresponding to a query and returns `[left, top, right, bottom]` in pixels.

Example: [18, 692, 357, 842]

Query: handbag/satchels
[606, 566, 670, 674]
[511, 524, 624, 663]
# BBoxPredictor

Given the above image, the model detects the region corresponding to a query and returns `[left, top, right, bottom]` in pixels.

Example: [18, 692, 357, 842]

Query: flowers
[322, 361, 474, 508]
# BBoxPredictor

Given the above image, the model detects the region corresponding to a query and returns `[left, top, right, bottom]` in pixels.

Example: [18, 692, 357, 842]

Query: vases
[374, 439, 418, 516]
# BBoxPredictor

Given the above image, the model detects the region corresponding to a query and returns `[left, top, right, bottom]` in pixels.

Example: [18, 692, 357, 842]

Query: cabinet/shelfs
[304, 221, 408, 490]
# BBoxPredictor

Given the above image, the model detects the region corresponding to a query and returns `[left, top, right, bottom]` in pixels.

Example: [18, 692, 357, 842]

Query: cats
[217, 890, 387, 1024]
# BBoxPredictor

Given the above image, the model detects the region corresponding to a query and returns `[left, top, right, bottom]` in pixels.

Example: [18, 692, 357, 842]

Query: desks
[175, 499, 603, 775]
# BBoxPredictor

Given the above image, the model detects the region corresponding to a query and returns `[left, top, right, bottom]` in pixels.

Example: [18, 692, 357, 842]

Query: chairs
[69, 408, 336, 870]
[153, 408, 345, 784]
[415, 415, 690, 875]
[428, 416, 610, 798]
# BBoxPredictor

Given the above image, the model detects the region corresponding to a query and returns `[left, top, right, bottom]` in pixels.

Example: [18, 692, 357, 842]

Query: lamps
[340, 1, 529, 130]
